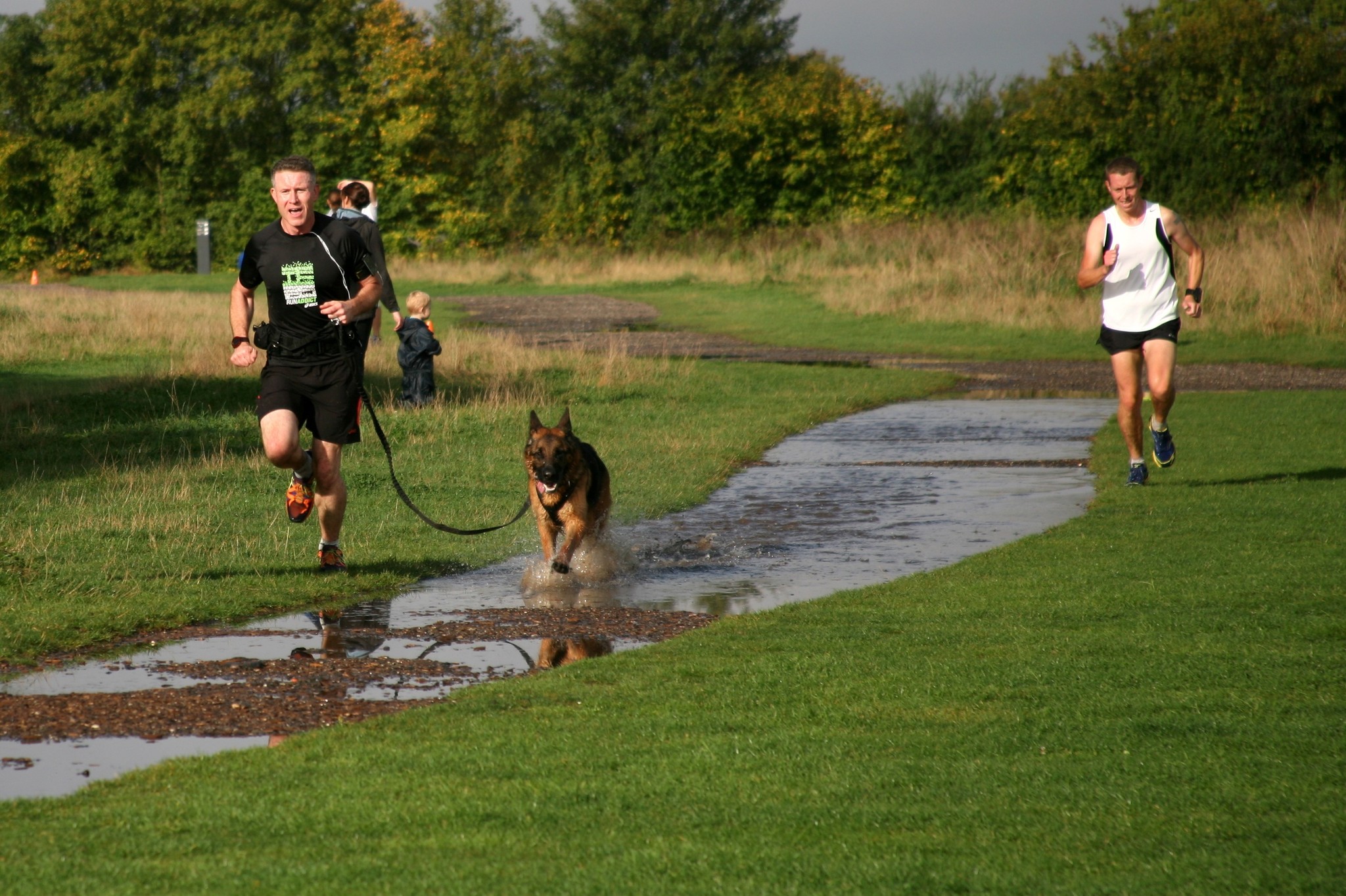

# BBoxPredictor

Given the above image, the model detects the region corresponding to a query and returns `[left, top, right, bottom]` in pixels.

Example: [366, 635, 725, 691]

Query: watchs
[232, 336, 250, 348]
[1186, 288, 1202, 302]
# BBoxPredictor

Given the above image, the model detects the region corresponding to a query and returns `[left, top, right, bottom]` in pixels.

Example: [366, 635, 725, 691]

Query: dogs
[522, 403, 615, 576]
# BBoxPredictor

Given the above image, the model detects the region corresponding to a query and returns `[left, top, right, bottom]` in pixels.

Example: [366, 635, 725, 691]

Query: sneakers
[316, 544, 348, 572]
[1124, 461, 1149, 487]
[1148, 414, 1176, 468]
[285, 449, 316, 523]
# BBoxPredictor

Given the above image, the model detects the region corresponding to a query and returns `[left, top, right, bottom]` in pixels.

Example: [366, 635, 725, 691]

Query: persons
[1076, 155, 1205, 489]
[230, 155, 384, 572]
[326, 177, 442, 410]
